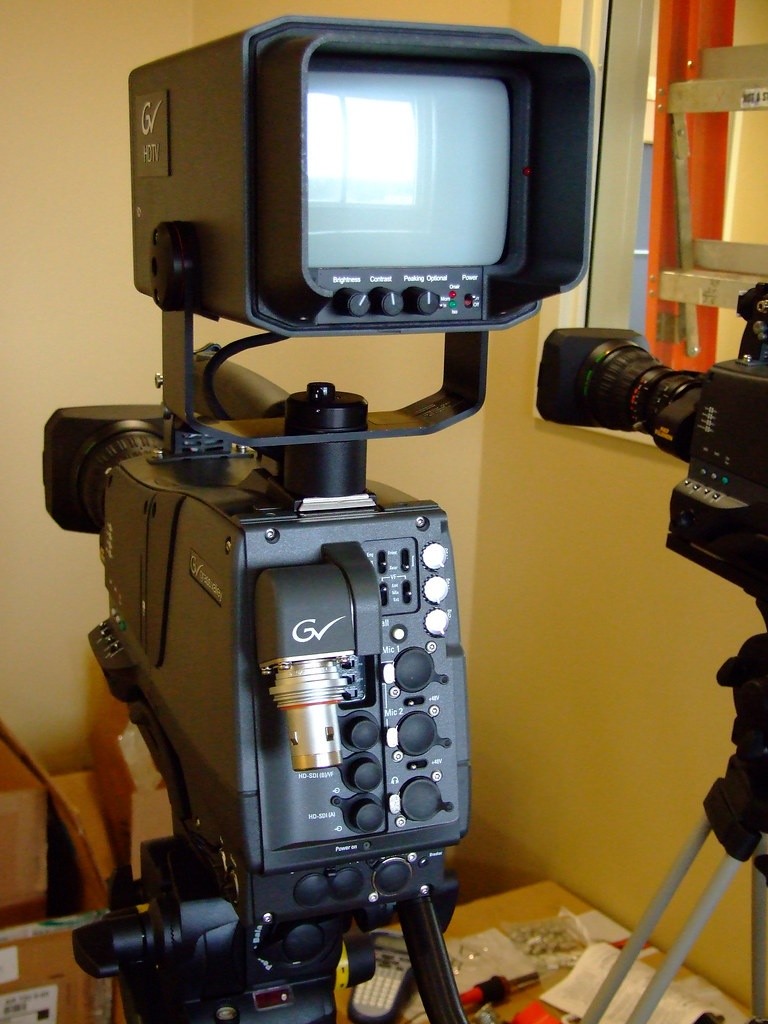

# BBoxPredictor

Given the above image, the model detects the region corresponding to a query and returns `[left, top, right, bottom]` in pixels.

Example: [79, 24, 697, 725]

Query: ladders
[644, 0, 768, 371]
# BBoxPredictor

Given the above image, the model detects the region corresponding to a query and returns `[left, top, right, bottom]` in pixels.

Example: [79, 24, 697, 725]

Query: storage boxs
[0, 724, 112, 1024]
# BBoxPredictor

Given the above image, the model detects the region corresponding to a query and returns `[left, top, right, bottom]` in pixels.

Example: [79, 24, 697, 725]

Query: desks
[334, 880, 768, 1024]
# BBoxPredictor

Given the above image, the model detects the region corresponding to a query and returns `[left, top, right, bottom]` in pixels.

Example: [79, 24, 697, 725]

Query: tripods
[582, 632, 768, 1024]
